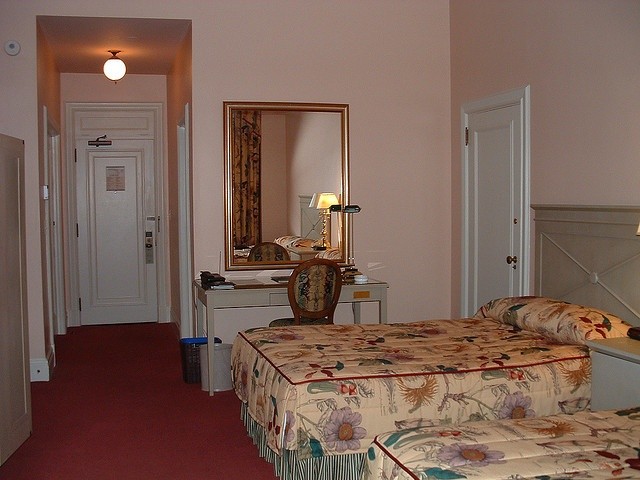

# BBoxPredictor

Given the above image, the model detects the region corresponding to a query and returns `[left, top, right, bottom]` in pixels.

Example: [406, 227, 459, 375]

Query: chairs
[248, 242, 290, 263]
[270, 259, 342, 326]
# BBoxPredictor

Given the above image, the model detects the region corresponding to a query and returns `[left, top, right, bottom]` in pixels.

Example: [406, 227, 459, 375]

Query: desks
[193, 277, 389, 397]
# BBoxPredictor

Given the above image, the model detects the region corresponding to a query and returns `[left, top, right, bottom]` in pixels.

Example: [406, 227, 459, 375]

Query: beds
[233, 194, 323, 263]
[231, 318, 591, 480]
[361, 411, 640, 480]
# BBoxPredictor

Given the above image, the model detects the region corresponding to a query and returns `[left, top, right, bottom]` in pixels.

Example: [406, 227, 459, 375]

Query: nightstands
[586, 338, 640, 412]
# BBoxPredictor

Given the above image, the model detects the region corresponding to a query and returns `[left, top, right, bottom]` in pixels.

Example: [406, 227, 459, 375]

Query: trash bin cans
[180, 338, 222, 383]
[199, 343, 234, 391]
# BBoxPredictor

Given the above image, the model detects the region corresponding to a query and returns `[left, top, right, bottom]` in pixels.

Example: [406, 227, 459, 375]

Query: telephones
[200, 270, 225, 283]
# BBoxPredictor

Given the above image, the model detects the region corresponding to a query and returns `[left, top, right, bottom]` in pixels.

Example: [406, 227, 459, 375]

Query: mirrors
[224, 101, 355, 271]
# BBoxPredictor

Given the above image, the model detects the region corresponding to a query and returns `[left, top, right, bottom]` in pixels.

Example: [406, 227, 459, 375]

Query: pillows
[274, 235, 315, 251]
[474, 296, 631, 346]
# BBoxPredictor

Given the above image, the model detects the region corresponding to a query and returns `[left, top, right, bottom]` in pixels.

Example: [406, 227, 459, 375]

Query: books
[270, 274, 291, 284]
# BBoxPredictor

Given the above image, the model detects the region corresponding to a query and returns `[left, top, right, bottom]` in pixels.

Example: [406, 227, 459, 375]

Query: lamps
[309, 192, 340, 250]
[103, 50, 127, 81]
[636, 225, 640, 235]
